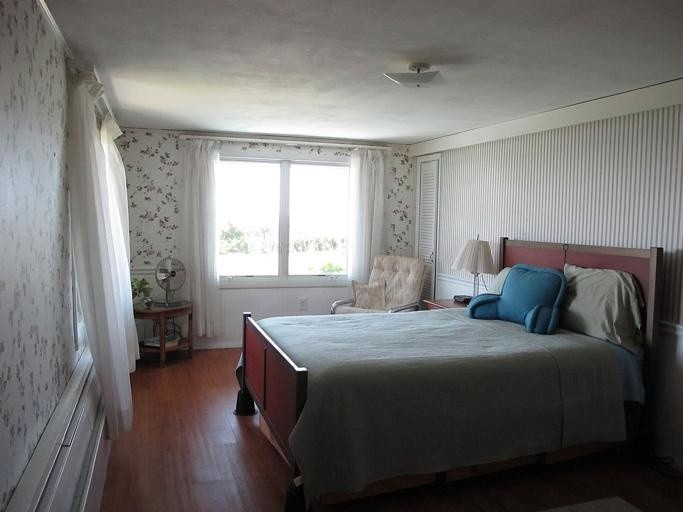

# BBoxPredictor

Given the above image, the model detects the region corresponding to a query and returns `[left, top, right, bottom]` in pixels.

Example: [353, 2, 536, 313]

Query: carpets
[537, 495, 642, 512]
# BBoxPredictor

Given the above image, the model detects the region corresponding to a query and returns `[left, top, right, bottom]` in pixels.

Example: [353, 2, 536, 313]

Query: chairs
[331, 255, 428, 314]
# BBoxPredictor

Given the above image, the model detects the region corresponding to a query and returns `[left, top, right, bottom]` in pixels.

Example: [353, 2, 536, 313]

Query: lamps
[451, 233, 497, 299]
[381, 63, 448, 90]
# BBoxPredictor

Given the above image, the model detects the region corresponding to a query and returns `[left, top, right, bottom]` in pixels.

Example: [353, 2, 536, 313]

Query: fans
[155, 257, 186, 308]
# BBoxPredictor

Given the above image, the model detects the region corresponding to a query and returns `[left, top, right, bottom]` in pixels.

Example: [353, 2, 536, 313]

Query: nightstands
[421, 298, 483, 309]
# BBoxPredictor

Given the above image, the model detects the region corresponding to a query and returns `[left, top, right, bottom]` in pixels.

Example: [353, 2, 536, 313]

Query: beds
[233, 238, 663, 512]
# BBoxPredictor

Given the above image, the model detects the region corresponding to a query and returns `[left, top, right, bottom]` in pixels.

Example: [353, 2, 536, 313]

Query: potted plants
[130, 277, 153, 310]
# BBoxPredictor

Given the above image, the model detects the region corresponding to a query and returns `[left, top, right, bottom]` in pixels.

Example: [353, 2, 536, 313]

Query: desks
[132, 300, 193, 369]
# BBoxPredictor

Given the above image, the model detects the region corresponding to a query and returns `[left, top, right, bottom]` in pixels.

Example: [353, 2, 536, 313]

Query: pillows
[558, 263, 646, 357]
[489, 266, 512, 294]
[350, 279, 388, 310]
[467, 263, 567, 335]
